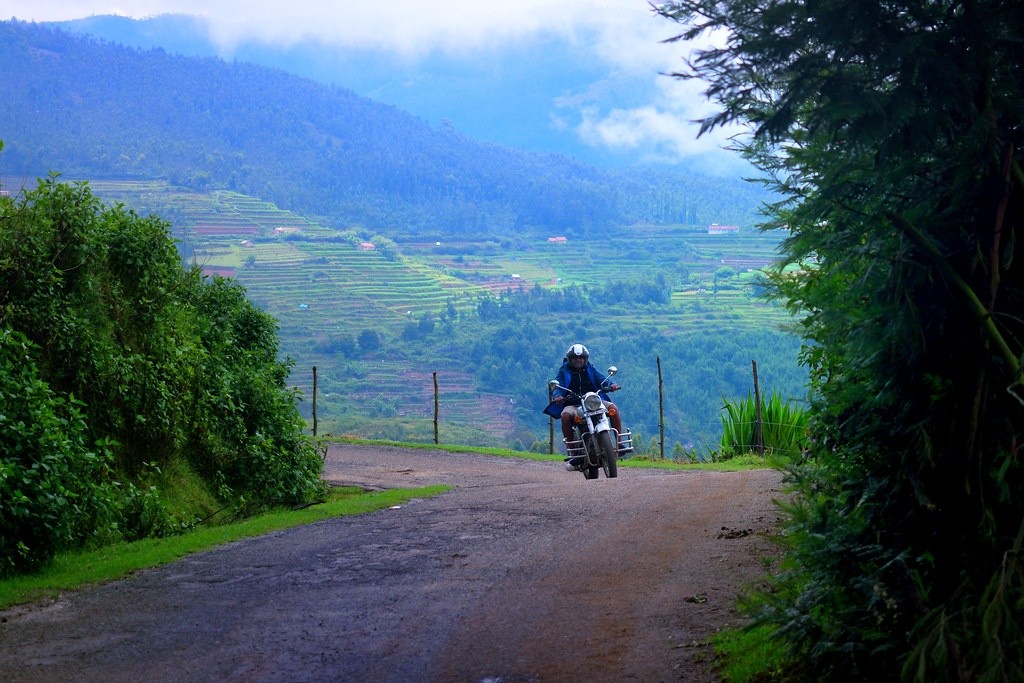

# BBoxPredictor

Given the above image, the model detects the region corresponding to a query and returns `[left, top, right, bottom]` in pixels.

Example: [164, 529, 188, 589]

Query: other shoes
[565, 454, 581, 471]
[619, 444, 633, 453]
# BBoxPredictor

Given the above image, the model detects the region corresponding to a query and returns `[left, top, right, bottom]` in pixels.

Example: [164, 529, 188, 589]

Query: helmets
[566, 344, 590, 357]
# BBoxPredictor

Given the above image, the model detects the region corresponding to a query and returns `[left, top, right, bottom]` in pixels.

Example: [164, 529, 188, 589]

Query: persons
[543, 343, 635, 467]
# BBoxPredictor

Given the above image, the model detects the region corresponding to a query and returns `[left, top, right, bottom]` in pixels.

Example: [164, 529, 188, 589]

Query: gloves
[611, 384, 618, 390]
[555, 396, 563, 404]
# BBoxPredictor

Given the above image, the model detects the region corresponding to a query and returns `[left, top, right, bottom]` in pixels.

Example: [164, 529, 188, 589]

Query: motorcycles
[547, 365, 635, 479]
